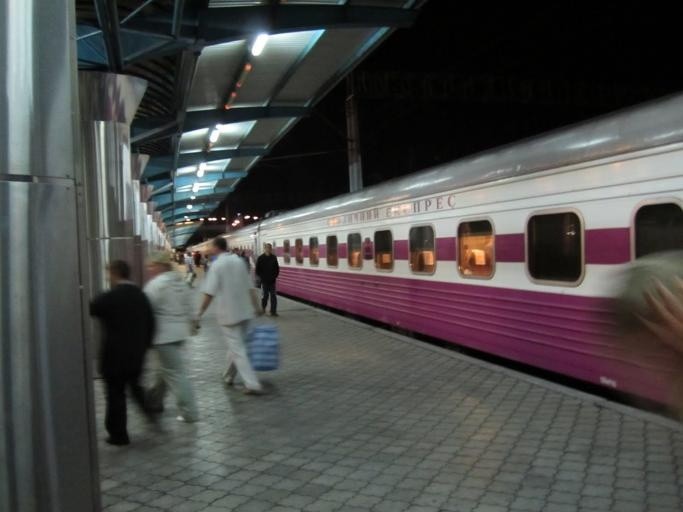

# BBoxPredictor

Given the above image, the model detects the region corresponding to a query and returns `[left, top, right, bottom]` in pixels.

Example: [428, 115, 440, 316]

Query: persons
[611, 249, 683, 387]
[90, 261, 197, 445]
[200, 237, 264, 397]
[254, 242, 279, 317]
[175, 248, 250, 287]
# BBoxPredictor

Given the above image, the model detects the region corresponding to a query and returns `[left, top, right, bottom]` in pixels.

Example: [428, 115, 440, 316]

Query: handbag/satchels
[245, 315, 280, 372]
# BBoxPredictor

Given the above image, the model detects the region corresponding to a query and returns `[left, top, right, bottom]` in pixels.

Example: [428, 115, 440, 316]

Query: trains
[187, 94, 683, 410]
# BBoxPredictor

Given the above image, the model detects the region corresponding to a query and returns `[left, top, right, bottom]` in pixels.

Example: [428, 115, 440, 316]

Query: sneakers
[103, 434, 131, 447]
[175, 413, 200, 423]
[218, 372, 268, 396]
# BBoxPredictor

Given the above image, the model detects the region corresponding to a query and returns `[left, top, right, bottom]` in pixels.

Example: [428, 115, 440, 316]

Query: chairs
[348, 249, 492, 276]
